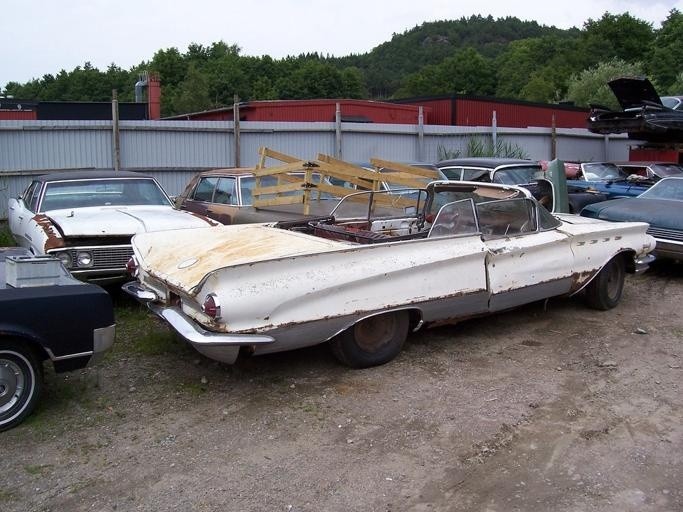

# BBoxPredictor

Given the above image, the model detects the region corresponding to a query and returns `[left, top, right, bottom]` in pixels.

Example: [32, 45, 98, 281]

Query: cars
[587, 74, 683, 147]
